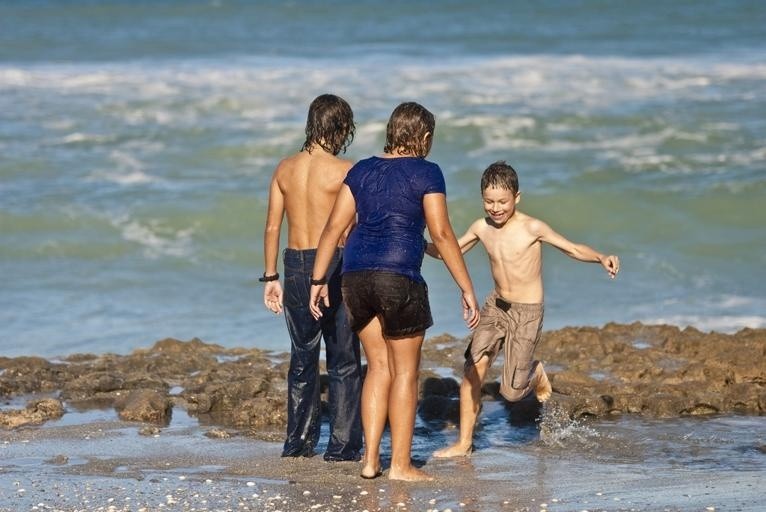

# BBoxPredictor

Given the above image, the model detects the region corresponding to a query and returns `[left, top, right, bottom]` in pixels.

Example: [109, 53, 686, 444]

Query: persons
[426, 160, 621, 458]
[312, 101, 481, 475]
[261, 92, 363, 464]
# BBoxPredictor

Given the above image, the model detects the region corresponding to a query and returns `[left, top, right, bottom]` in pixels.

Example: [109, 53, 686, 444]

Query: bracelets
[423, 237, 428, 254]
[259, 273, 280, 281]
[308, 280, 326, 285]
